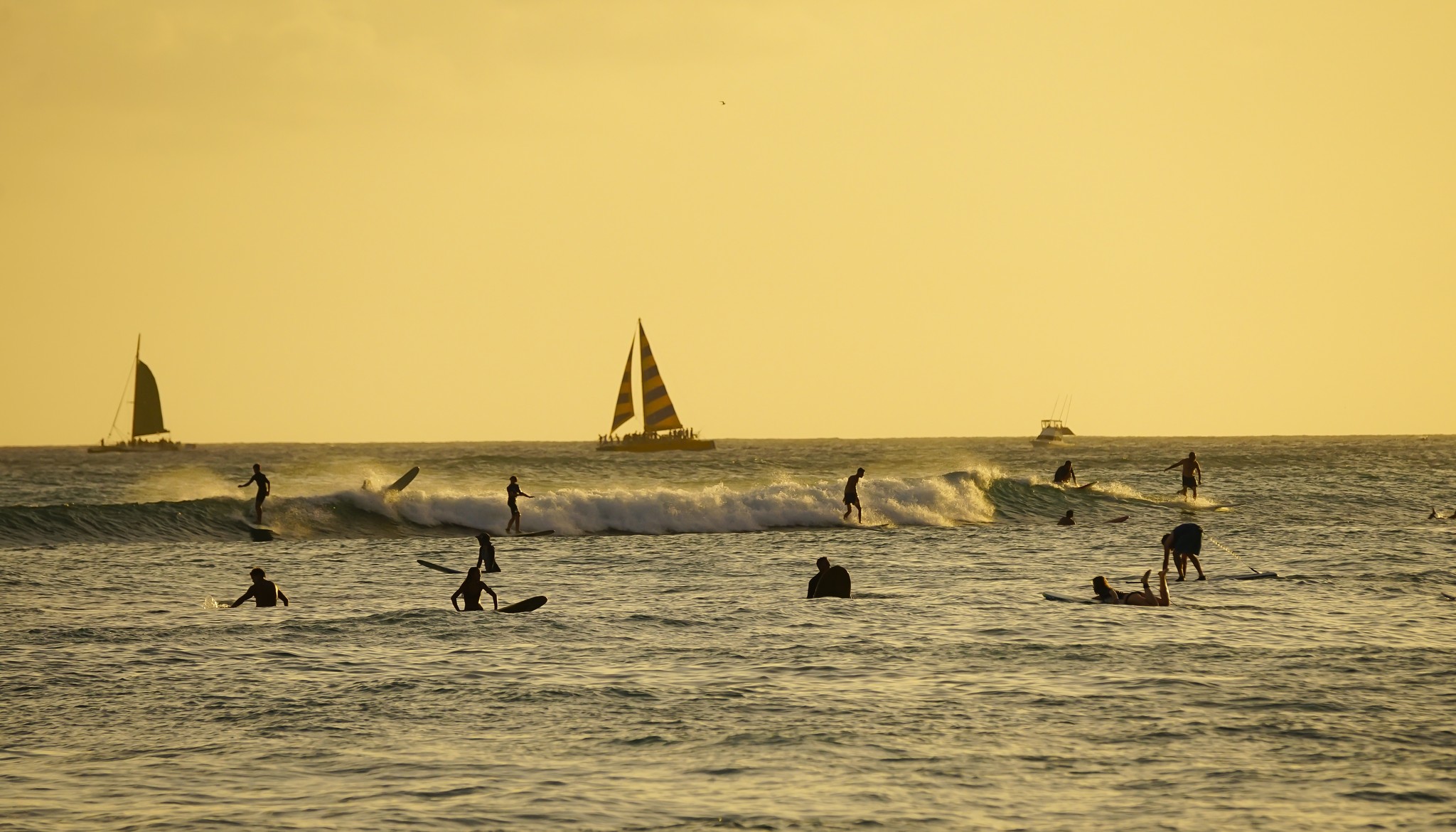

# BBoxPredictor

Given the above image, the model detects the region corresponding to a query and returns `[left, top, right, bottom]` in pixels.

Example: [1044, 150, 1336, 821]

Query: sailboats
[595, 318, 717, 451]
[87, 333, 179, 454]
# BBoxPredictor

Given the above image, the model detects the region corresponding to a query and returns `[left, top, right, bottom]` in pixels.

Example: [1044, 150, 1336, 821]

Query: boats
[1029, 392, 1076, 448]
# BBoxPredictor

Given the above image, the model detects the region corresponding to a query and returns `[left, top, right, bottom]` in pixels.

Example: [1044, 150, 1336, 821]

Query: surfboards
[386, 466, 420, 492]
[1212, 502, 1246, 508]
[490, 596, 548, 614]
[1077, 481, 1099, 490]
[1042, 590, 1102, 605]
[490, 529, 555, 539]
[239, 518, 280, 537]
[839, 523, 889, 530]
[1106, 515, 1130, 523]
[812, 565, 852, 599]
[1169, 571, 1280, 582]
[417, 560, 492, 576]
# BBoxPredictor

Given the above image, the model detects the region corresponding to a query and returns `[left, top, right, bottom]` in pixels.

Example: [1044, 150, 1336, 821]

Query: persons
[1056, 510, 1076, 525]
[238, 463, 270, 525]
[1164, 452, 1202, 505]
[1161, 523, 1207, 582]
[1427, 508, 1456, 519]
[806, 556, 831, 599]
[843, 467, 865, 524]
[101, 438, 181, 446]
[475, 533, 501, 573]
[505, 476, 536, 534]
[451, 567, 498, 612]
[1053, 460, 1078, 487]
[599, 427, 694, 444]
[230, 568, 289, 608]
[1091, 568, 1170, 607]
